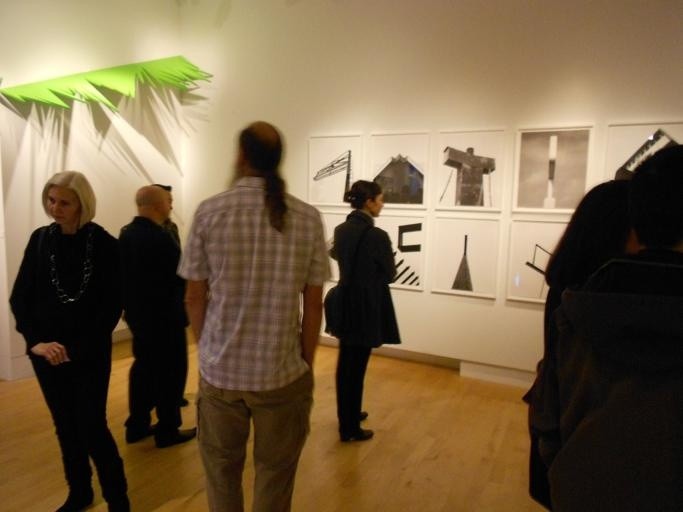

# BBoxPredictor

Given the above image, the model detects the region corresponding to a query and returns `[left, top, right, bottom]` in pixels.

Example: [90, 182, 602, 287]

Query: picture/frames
[606, 121, 682, 182]
[304, 117, 594, 305]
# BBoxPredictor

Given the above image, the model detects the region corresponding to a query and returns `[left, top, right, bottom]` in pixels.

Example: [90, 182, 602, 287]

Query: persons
[150, 183, 188, 408]
[177, 121, 332, 512]
[8, 171, 130, 512]
[118, 186, 197, 448]
[522, 141, 679, 511]
[324, 180, 401, 443]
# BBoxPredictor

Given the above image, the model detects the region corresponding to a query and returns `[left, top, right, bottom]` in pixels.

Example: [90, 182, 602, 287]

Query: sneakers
[358, 411, 368, 422]
[125, 423, 158, 443]
[106, 494, 131, 512]
[53, 487, 94, 512]
[154, 427, 198, 448]
[340, 428, 374, 443]
